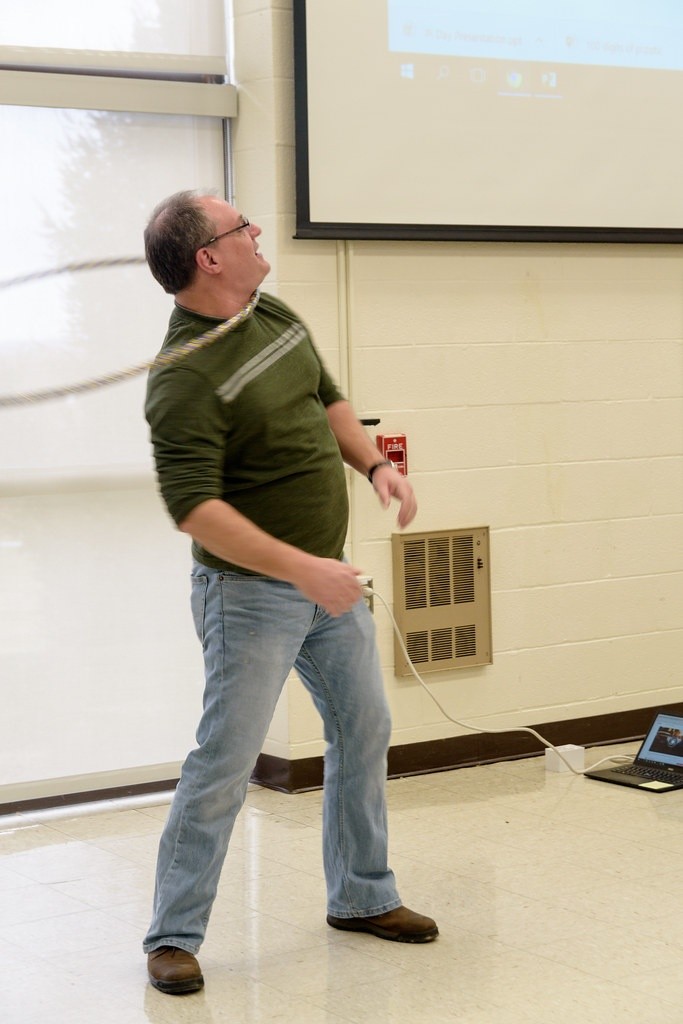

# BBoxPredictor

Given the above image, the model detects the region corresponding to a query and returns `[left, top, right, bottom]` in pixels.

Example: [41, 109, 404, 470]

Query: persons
[137, 185, 443, 998]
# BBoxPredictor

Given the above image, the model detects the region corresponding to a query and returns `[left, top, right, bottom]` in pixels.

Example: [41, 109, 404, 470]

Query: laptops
[584, 712, 683, 793]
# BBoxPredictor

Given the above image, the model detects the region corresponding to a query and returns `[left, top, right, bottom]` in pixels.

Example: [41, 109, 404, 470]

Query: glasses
[201, 217, 250, 247]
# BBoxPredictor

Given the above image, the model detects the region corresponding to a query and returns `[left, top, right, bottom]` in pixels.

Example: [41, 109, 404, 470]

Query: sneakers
[327, 906, 439, 943]
[147, 945, 204, 993]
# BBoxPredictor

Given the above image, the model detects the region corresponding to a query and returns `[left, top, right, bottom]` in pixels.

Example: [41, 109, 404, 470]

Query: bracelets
[367, 459, 400, 485]
